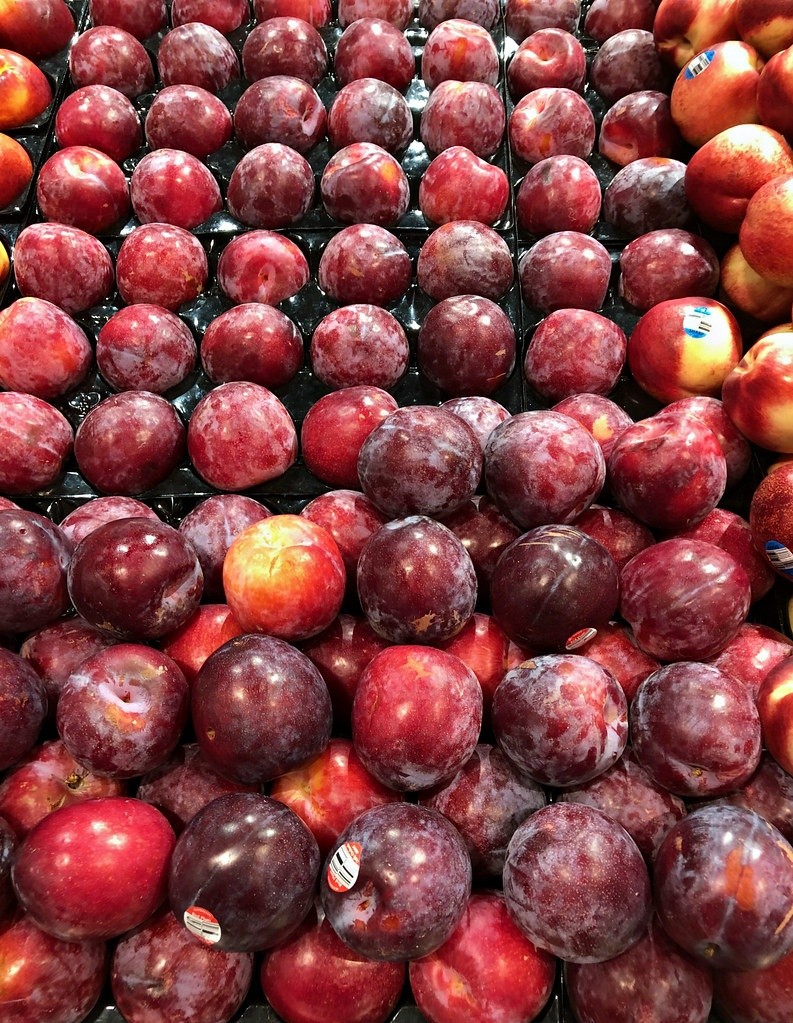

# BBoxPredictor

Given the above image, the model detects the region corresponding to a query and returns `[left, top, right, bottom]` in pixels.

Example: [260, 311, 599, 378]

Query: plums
[0, 0, 793, 1023]
